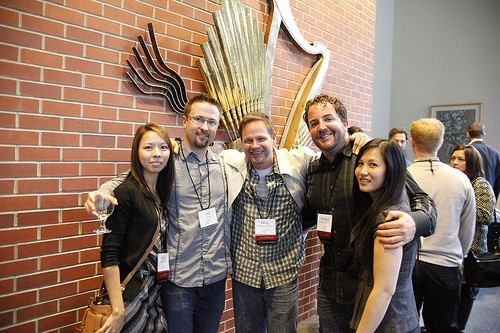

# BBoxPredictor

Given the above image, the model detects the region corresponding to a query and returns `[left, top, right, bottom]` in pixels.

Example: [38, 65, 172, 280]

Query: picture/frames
[428, 101, 484, 163]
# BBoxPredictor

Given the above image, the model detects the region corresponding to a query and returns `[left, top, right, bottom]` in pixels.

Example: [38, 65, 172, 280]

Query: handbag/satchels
[487, 221, 500, 252]
[82, 302, 112, 333]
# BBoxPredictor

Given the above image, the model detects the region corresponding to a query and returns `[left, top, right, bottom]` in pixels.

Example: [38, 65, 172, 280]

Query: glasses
[450, 158, 466, 164]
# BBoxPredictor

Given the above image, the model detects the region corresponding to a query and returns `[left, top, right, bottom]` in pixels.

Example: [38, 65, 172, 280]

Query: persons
[94, 122, 172, 333]
[401, 117, 477, 333]
[302, 93, 437, 333]
[339, 137, 421, 333]
[447, 142, 496, 333]
[463, 122, 500, 220]
[382, 127, 415, 168]
[85, 93, 252, 333]
[164, 111, 373, 333]
